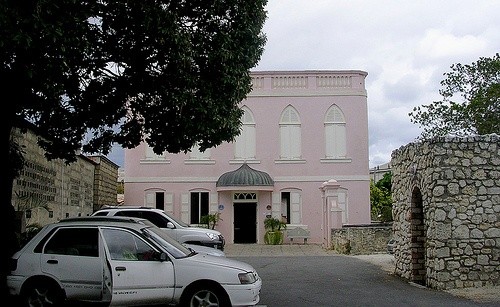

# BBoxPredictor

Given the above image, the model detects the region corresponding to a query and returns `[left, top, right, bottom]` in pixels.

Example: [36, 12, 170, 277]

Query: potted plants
[264, 217, 286, 244]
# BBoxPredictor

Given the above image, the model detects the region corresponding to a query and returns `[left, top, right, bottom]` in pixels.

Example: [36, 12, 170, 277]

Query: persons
[105, 233, 140, 261]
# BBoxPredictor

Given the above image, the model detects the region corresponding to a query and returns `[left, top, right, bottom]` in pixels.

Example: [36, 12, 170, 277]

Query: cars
[6, 221, 262, 307]
[59, 216, 224, 257]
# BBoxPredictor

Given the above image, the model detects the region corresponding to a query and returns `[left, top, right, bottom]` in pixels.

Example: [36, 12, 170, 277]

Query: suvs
[90, 205, 225, 251]
